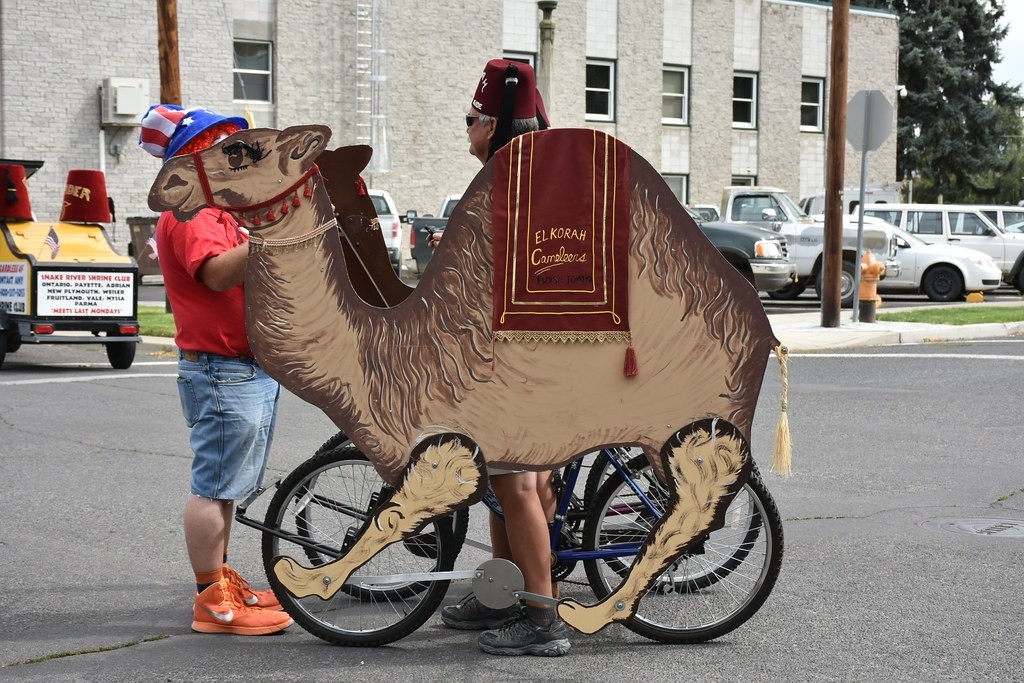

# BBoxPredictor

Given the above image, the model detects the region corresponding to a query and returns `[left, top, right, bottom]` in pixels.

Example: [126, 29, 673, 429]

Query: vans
[798, 189, 1024, 291]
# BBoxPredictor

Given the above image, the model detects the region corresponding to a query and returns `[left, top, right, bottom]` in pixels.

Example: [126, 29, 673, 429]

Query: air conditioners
[99, 74, 149, 127]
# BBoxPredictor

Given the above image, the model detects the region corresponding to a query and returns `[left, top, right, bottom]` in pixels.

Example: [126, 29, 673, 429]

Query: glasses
[466, 114, 479, 126]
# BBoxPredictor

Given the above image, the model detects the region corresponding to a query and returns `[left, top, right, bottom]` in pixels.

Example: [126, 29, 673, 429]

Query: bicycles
[260, 430, 783, 647]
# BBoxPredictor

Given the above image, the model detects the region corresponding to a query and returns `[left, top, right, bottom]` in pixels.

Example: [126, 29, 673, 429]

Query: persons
[425, 60, 571, 658]
[138, 104, 293, 636]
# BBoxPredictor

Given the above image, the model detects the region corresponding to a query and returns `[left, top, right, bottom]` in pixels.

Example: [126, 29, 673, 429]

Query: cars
[807, 215, 1002, 302]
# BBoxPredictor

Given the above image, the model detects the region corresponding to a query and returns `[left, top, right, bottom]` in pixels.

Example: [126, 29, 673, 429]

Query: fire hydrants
[858, 248, 885, 323]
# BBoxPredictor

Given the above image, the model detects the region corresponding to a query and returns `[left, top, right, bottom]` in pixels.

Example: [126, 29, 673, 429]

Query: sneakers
[477, 608, 571, 657]
[191, 583, 294, 635]
[193, 562, 285, 611]
[441, 591, 522, 630]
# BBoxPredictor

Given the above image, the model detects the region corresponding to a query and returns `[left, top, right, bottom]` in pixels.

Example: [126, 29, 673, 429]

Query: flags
[44, 229, 59, 259]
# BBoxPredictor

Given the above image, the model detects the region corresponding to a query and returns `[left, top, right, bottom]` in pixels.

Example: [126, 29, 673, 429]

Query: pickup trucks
[367, 190, 416, 277]
[720, 186, 901, 310]
[410, 196, 797, 294]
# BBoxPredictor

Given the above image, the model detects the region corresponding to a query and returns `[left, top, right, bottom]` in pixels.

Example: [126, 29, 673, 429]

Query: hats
[139, 104, 249, 161]
[471, 60, 551, 131]
